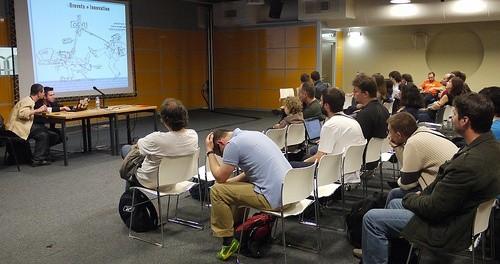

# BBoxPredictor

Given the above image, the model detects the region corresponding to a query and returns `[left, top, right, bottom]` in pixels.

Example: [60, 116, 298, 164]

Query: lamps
[269, 0, 284, 19]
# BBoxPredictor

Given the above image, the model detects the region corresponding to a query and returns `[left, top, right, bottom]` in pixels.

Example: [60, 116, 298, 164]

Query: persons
[205, 128, 294, 261]
[272, 71, 500, 209]
[352, 92, 500, 264]
[119, 98, 199, 191]
[33, 86, 77, 147]
[4, 84, 55, 167]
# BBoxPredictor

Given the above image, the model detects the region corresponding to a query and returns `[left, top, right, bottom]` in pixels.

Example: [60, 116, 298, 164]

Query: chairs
[0, 115, 32, 172]
[175, 88, 395, 264]
[443, 105, 453, 128]
[129, 146, 205, 247]
[417, 196, 496, 264]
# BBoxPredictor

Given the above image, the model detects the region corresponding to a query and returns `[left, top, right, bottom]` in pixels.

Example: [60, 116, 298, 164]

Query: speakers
[268, 1, 284, 19]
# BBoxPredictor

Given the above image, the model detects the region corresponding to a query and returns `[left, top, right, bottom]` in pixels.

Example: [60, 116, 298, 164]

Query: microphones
[93, 86, 107, 98]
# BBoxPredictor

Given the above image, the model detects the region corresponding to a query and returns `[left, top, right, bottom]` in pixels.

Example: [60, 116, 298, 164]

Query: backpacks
[342, 191, 388, 247]
[118, 188, 158, 233]
[229, 210, 277, 259]
[184, 178, 215, 202]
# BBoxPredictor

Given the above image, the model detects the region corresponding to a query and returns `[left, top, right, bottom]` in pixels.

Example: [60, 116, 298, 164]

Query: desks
[40, 104, 157, 167]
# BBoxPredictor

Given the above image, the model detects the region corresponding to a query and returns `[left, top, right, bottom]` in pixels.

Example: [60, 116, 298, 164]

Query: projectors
[246, 0, 265, 6]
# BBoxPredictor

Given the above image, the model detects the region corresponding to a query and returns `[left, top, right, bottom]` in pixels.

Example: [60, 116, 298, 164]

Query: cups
[47, 107, 52, 113]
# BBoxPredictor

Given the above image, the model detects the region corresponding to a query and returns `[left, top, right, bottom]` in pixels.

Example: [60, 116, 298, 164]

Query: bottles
[443, 119, 448, 134]
[95, 96, 100, 109]
[448, 116, 453, 131]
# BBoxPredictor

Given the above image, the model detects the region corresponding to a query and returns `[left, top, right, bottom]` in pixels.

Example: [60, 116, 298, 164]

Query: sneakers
[215, 238, 240, 261]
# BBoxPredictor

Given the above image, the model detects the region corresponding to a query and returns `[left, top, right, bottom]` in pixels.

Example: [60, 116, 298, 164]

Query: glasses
[353, 89, 365, 94]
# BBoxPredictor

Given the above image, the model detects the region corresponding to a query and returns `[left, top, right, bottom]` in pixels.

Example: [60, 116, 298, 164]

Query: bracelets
[207, 150, 214, 157]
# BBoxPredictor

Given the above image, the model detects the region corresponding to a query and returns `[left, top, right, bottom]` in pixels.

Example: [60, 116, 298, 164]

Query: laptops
[67, 98, 92, 112]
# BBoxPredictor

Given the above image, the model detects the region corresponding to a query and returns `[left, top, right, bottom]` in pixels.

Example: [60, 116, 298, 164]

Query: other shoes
[352, 248, 363, 258]
[32, 158, 54, 165]
